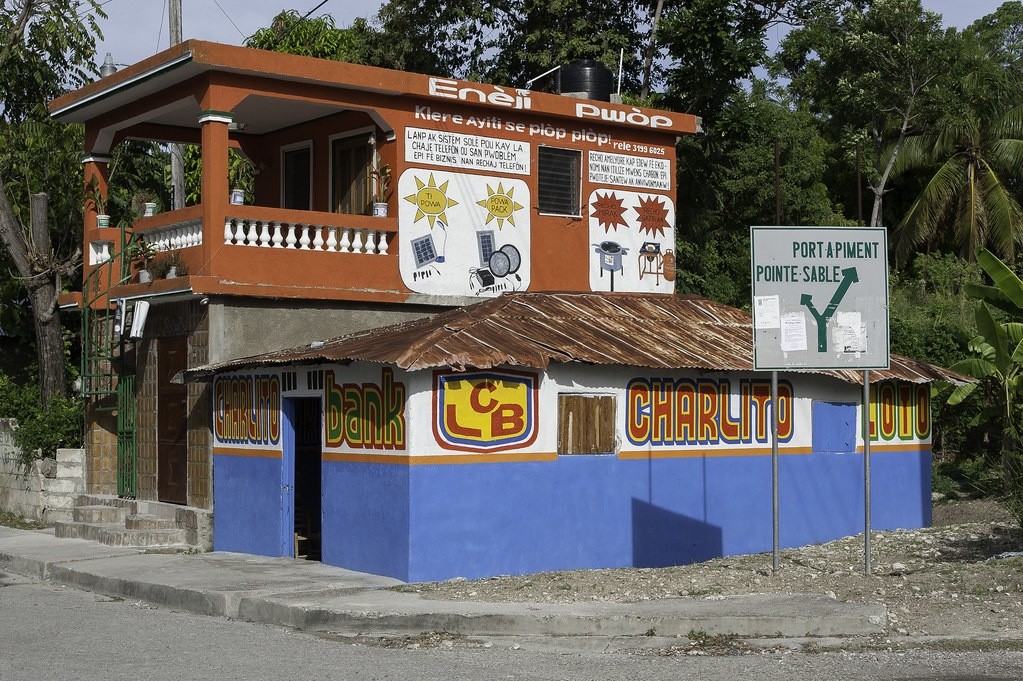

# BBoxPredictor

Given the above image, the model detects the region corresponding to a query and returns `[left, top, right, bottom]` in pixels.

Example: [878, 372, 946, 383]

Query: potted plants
[362, 152, 393, 218]
[149, 243, 188, 279]
[83, 173, 112, 228]
[229, 158, 251, 205]
[128, 241, 158, 283]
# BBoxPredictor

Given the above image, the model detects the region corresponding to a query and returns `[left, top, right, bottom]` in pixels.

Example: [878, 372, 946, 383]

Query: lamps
[368, 133, 376, 145]
[99, 53, 129, 78]
